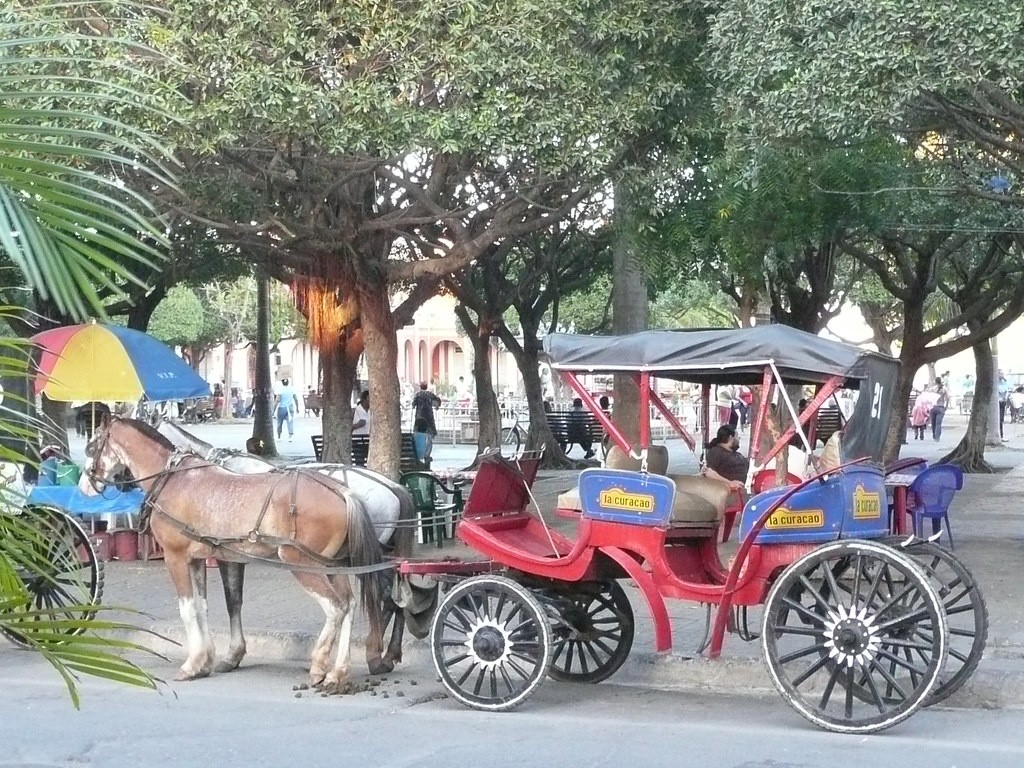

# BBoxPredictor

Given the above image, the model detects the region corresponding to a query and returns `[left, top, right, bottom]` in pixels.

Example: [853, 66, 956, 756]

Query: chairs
[398, 471, 462, 548]
[753, 470, 803, 496]
[892, 463, 965, 547]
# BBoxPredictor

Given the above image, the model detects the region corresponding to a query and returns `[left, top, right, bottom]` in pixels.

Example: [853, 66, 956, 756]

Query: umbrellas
[28, 319, 214, 534]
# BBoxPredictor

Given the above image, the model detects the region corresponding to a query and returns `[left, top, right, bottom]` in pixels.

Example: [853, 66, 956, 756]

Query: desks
[419, 470, 478, 512]
[23, 484, 149, 565]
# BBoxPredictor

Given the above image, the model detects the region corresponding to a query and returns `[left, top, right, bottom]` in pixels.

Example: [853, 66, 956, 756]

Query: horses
[78, 414, 414, 689]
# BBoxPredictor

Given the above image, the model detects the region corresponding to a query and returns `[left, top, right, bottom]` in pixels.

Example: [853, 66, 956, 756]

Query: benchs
[310, 432, 433, 474]
[545, 411, 611, 459]
[738, 466, 890, 546]
[554, 444, 732, 531]
[184, 400, 216, 424]
[794, 405, 841, 444]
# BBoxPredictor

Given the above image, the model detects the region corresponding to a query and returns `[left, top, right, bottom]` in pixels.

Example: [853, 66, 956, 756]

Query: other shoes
[584, 451, 595, 459]
[289, 438, 293, 441]
[915, 434, 918, 438]
[1001, 438, 1009, 442]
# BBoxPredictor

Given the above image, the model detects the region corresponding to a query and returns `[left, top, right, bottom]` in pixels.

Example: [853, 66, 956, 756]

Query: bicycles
[497, 408, 575, 458]
[0, 500, 106, 655]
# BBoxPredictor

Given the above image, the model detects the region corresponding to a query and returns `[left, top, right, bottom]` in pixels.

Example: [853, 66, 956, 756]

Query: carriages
[76, 321, 992, 738]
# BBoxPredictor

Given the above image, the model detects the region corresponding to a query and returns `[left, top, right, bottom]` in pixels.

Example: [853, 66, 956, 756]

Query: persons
[700, 424, 759, 491]
[657, 385, 753, 434]
[544, 396, 567, 455]
[963, 375, 974, 394]
[913, 371, 950, 442]
[351, 390, 371, 467]
[798, 389, 857, 447]
[412, 381, 442, 462]
[591, 397, 612, 440]
[497, 392, 514, 418]
[400, 377, 467, 402]
[273, 378, 300, 443]
[177, 379, 256, 417]
[566, 398, 596, 458]
[998, 376, 1024, 441]
[75, 401, 134, 440]
[308, 385, 321, 417]
